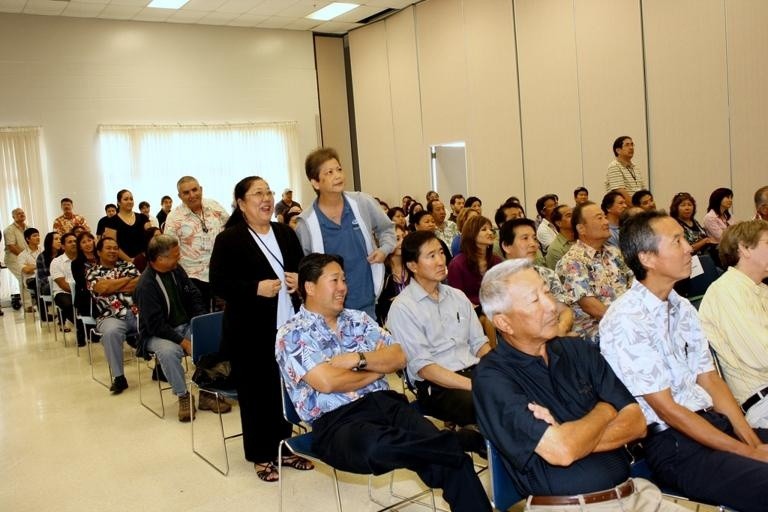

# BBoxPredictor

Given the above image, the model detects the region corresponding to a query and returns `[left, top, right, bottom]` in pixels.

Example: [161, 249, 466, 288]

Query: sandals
[254, 463, 280, 482]
[271, 455, 314, 471]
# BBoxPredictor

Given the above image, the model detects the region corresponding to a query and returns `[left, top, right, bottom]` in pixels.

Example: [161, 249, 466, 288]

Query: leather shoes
[24, 307, 36, 312]
[110, 377, 129, 391]
[152, 367, 167, 381]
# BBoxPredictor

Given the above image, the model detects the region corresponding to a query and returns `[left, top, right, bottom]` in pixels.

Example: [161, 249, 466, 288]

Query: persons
[597, 209, 767, 510]
[209, 175, 315, 482]
[274, 253, 494, 511]
[1, 175, 232, 421]
[474, 256, 728, 511]
[373, 186, 768, 379]
[274, 189, 304, 231]
[296, 148, 397, 323]
[604, 135, 645, 205]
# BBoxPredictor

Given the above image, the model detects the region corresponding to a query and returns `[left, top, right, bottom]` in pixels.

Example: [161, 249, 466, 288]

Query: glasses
[677, 194, 689, 197]
[243, 192, 274, 198]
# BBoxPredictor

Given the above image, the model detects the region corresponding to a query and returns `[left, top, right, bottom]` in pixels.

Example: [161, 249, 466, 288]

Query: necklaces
[625, 165, 637, 186]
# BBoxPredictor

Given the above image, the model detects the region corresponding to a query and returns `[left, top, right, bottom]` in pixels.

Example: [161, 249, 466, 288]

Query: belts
[414, 366, 479, 388]
[627, 408, 714, 458]
[527, 481, 635, 505]
[739, 386, 766, 417]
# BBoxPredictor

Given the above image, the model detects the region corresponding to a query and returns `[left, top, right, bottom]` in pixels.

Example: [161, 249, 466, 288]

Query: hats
[281, 188, 293, 197]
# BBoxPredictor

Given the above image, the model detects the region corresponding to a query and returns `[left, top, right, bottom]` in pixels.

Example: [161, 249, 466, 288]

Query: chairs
[378, 295, 490, 511]
[271, 358, 438, 511]
[127, 284, 194, 419]
[475, 418, 530, 512]
[183, 311, 311, 478]
[15, 272, 191, 421]
[14, 266, 533, 510]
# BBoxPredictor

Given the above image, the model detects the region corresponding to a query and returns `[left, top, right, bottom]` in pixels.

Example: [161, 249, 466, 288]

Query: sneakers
[198, 390, 232, 413]
[177, 394, 197, 422]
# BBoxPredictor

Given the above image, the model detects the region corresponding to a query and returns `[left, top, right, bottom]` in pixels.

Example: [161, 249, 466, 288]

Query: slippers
[60, 327, 71, 332]
